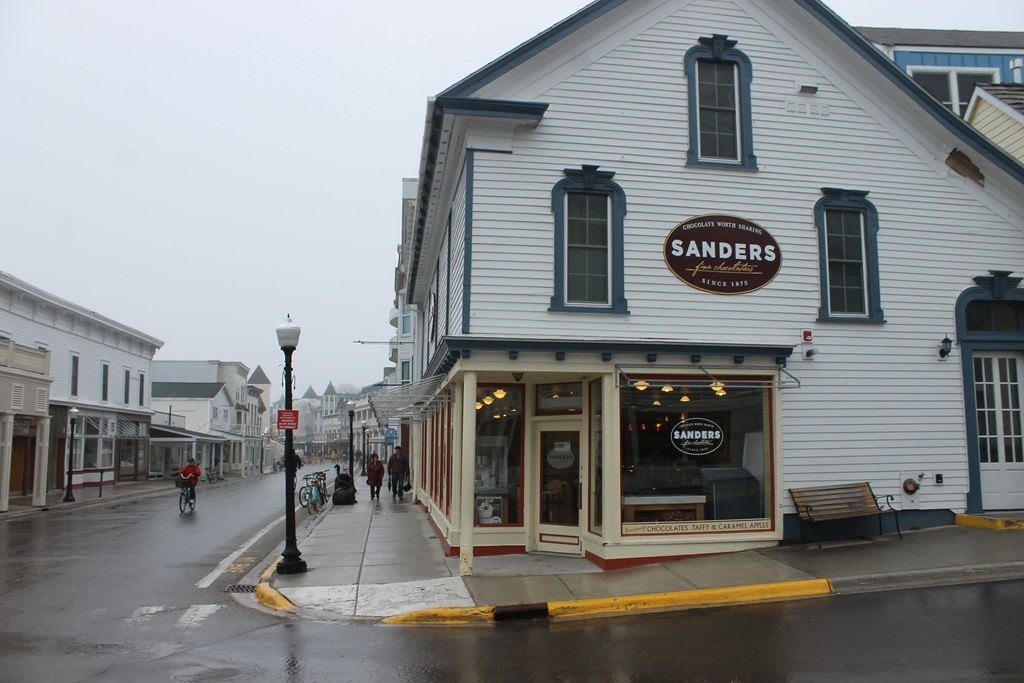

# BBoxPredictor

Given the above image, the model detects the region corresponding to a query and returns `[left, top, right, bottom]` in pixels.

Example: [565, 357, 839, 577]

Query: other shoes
[399, 496, 402, 500]
[392, 493, 396, 499]
[189, 499, 194, 504]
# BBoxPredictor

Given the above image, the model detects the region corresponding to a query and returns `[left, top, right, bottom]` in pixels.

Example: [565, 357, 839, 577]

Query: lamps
[936, 332, 953, 361]
[806, 347, 821, 358]
[476, 388, 508, 419]
[679, 393, 692, 403]
[707, 380, 727, 396]
[661, 385, 675, 393]
[652, 400, 661, 406]
[511, 373, 525, 382]
[633, 380, 651, 391]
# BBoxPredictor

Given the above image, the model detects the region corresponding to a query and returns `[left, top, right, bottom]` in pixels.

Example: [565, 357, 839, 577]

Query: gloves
[177, 471, 181, 475]
[193, 472, 198, 477]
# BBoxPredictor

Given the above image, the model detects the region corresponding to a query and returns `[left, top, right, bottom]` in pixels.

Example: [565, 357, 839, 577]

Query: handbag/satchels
[403, 475, 412, 492]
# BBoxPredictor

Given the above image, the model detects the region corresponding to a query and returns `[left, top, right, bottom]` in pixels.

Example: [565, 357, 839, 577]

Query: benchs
[787, 480, 904, 550]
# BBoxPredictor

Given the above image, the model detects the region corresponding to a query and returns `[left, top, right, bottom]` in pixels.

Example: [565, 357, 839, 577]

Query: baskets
[175, 478, 189, 487]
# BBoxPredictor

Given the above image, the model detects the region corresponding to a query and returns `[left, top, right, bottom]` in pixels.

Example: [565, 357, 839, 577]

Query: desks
[622, 463, 707, 521]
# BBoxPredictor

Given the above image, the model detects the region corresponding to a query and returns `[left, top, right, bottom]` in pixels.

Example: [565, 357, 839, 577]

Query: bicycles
[175, 471, 196, 514]
[269, 464, 283, 474]
[298, 469, 329, 508]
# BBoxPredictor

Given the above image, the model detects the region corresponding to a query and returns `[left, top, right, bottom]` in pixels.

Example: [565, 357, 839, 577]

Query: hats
[394, 446, 402, 449]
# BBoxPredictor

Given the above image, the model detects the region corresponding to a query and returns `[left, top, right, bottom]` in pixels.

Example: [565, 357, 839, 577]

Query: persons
[179, 458, 201, 504]
[367, 454, 384, 500]
[319, 448, 362, 464]
[293, 448, 301, 476]
[388, 446, 409, 500]
[370, 451, 378, 462]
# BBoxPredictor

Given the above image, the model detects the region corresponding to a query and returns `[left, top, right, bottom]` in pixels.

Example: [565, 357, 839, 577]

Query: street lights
[276, 314, 308, 574]
[62, 405, 79, 503]
[345, 396, 357, 493]
[360, 420, 367, 476]
[365, 429, 370, 464]
